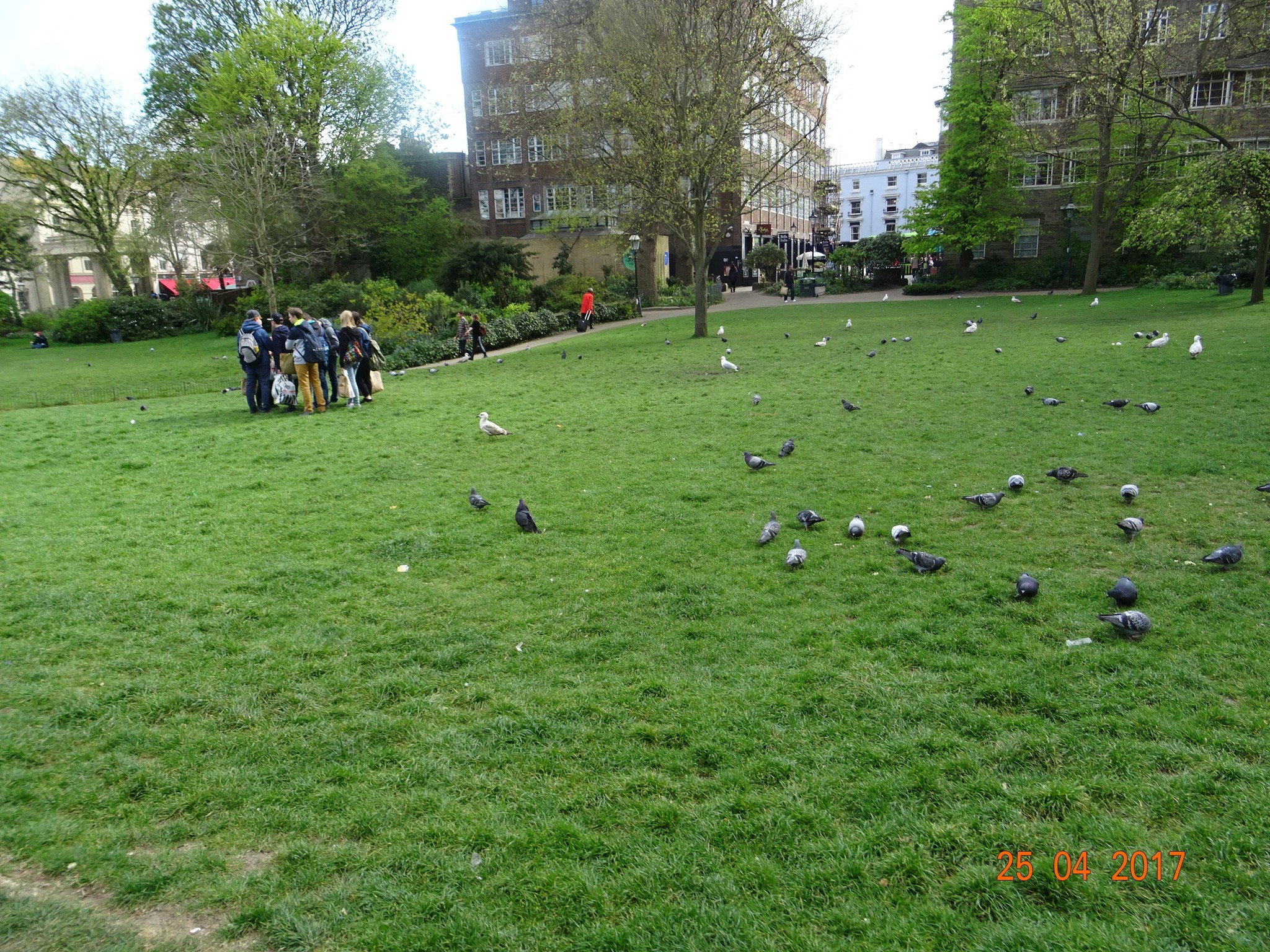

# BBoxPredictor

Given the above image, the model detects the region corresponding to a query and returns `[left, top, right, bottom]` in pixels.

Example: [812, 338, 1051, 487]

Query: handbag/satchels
[337, 368, 355, 398]
[279, 353, 296, 374]
[270, 373, 298, 406]
[780, 286, 788, 295]
[343, 329, 364, 364]
[369, 370, 384, 394]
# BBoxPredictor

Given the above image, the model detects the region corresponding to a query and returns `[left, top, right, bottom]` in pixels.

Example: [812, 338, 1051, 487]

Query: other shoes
[270, 402, 278, 408]
[784, 300, 788, 303]
[298, 411, 313, 416]
[346, 398, 372, 408]
[251, 406, 266, 413]
[791, 299, 796, 302]
[283, 407, 296, 413]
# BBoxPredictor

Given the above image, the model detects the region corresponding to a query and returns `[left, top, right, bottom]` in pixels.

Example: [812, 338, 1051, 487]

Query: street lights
[791, 221, 798, 269]
[809, 210, 818, 277]
[1059, 183, 1076, 290]
[628, 227, 644, 318]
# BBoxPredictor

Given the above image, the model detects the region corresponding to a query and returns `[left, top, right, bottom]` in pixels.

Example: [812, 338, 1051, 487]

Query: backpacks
[479, 326, 486, 336]
[327, 326, 341, 356]
[312, 327, 329, 369]
[294, 326, 325, 362]
[239, 328, 263, 365]
[357, 326, 386, 371]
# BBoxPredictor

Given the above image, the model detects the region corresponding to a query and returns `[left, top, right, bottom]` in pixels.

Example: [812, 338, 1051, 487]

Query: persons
[911, 254, 942, 278]
[728, 264, 738, 292]
[581, 287, 595, 329]
[465, 314, 488, 361]
[453, 311, 469, 356]
[237, 307, 372, 416]
[30, 331, 49, 348]
[783, 265, 796, 302]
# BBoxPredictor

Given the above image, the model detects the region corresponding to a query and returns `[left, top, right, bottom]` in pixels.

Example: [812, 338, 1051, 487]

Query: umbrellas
[797, 251, 826, 269]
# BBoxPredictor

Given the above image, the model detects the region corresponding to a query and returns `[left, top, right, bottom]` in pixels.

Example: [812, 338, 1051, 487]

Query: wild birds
[720, 356, 740, 373]
[1011, 295, 1021, 303]
[962, 323, 977, 335]
[515, 499, 539, 534]
[883, 293, 888, 302]
[721, 337, 728, 343]
[476, 412, 513, 441]
[717, 325, 724, 335]
[1189, 335, 1202, 358]
[1143, 332, 1169, 351]
[458, 351, 470, 363]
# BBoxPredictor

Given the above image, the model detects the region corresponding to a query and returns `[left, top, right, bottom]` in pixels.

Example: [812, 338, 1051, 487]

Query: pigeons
[642, 321, 646, 326]
[390, 362, 449, 376]
[578, 355, 582, 359]
[726, 348, 731, 355]
[665, 338, 671, 345]
[562, 349, 566, 359]
[496, 359, 504, 363]
[742, 290, 1270, 642]
[469, 487, 492, 511]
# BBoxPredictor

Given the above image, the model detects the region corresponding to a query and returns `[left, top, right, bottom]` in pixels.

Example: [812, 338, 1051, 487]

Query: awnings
[159, 277, 235, 296]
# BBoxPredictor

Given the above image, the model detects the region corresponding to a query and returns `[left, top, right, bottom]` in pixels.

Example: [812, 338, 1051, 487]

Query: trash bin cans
[800, 277, 815, 298]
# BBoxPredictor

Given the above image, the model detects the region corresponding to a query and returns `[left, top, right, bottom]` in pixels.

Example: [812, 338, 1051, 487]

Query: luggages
[576, 310, 592, 332]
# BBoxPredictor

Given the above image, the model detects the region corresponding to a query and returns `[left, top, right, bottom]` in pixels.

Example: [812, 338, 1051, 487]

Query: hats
[244, 309, 260, 322]
[267, 313, 283, 323]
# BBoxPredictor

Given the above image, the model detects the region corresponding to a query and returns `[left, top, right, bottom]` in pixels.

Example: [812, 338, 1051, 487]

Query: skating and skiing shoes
[240, 378, 257, 395]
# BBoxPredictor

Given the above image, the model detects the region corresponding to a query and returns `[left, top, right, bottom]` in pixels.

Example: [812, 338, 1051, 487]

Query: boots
[468, 353, 475, 360]
[483, 352, 487, 358]
[590, 323, 594, 329]
